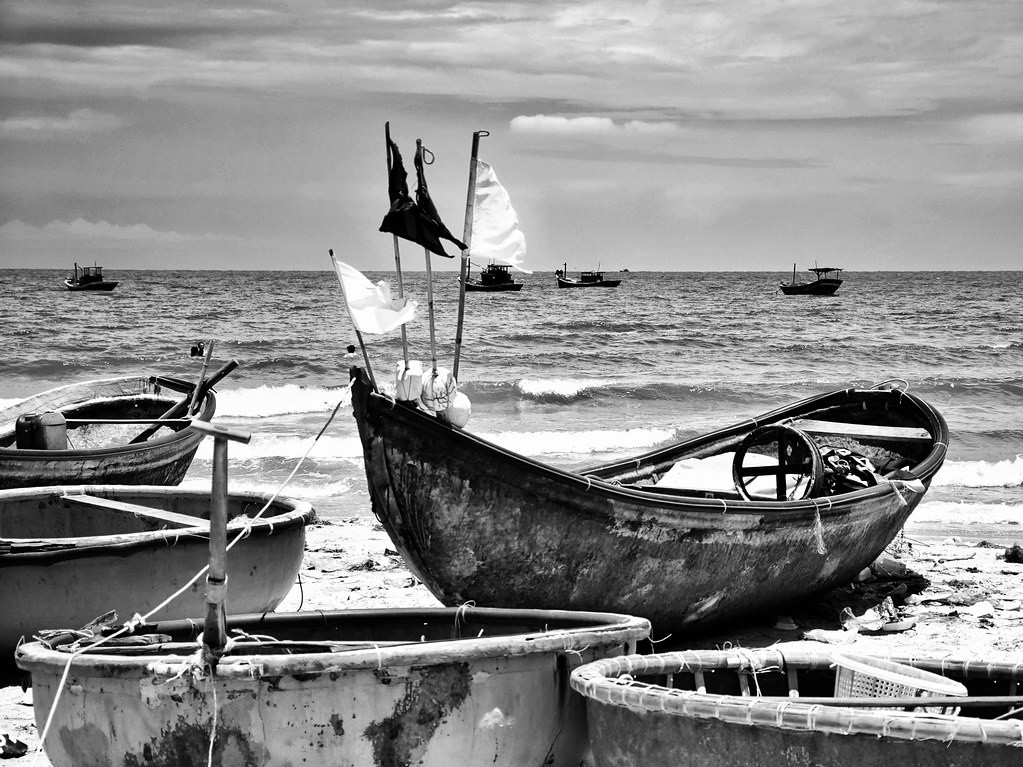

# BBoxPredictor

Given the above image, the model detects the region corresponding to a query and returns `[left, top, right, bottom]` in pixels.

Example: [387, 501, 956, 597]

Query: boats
[0, 484, 315, 656]
[347, 365, 948, 635]
[556, 263, 622, 287]
[13, 600, 652, 767]
[64, 262, 119, 291]
[0, 375, 216, 490]
[570, 646, 1023, 767]
[781, 264, 843, 296]
[457, 258, 524, 292]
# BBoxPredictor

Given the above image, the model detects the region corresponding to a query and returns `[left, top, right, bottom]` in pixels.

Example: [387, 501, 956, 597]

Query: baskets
[826, 651, 967, 717]
